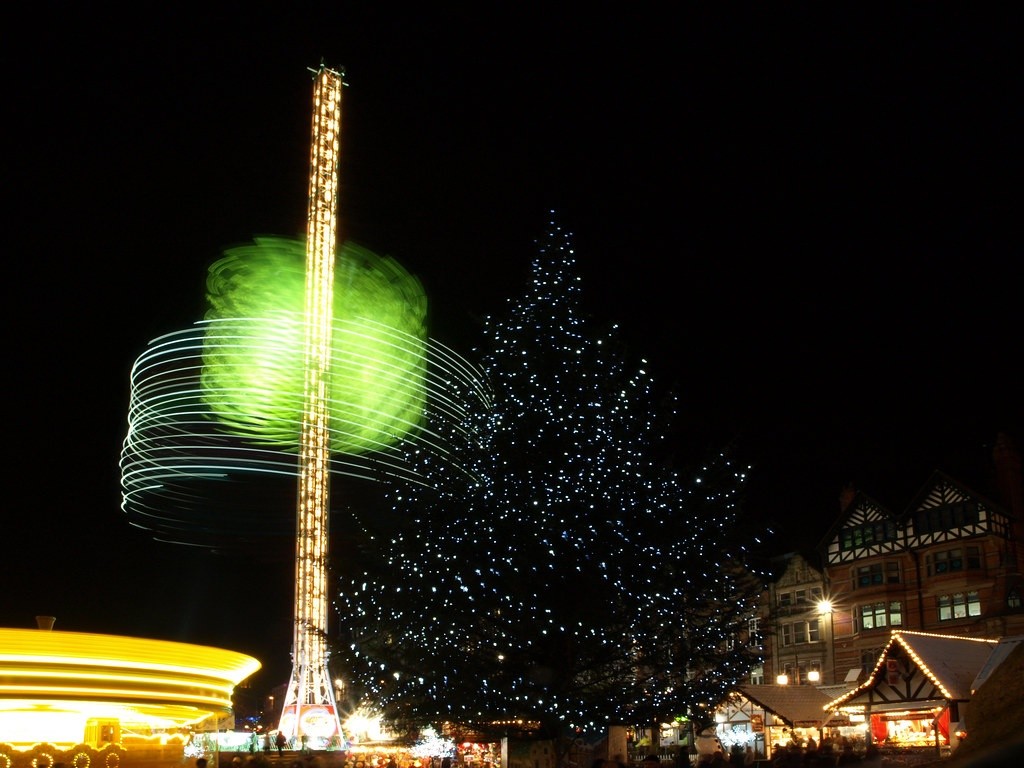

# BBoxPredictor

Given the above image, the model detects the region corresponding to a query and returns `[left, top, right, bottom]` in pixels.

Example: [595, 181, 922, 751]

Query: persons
[634, 734, 650, 757]
[248, 728, 258, 754]
[275, 731, 286, 757]
[261, 730, 272, 754]
[593, 732, 891, 768]
